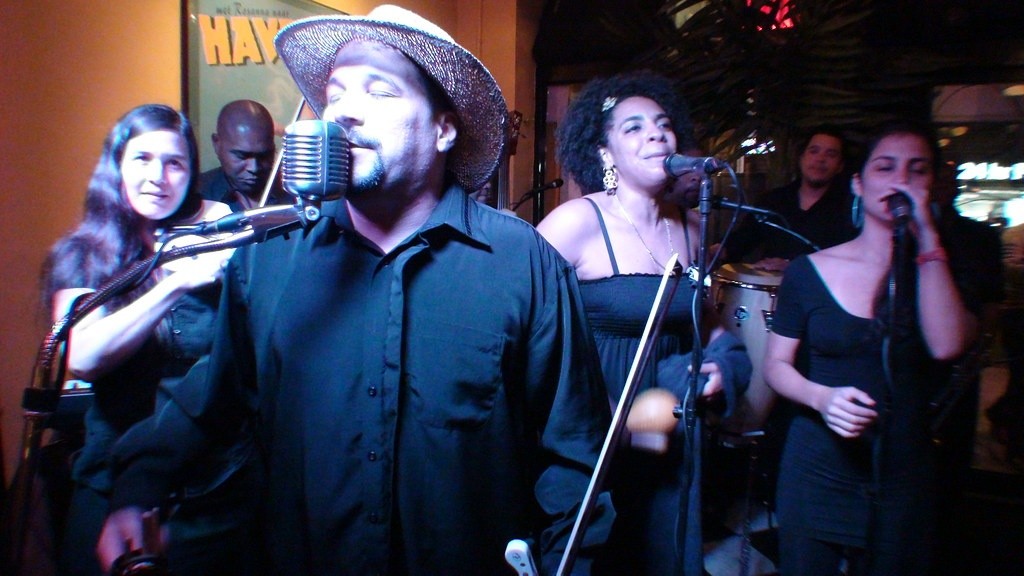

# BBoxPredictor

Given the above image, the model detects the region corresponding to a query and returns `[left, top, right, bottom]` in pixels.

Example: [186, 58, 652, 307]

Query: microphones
[194, 120, 351, 236]
[710, 194, 773, 217]
[661, 152, 728, 179]
[525, 179, 564, 195]
[887, 192, 911, 223]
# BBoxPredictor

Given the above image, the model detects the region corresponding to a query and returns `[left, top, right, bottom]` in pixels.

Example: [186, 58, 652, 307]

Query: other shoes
[985, 405, 1010, 425]
[1006, 440, 1024, 459]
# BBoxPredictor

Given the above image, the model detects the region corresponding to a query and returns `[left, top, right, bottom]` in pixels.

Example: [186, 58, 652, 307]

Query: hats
[274, 4, 506, 193]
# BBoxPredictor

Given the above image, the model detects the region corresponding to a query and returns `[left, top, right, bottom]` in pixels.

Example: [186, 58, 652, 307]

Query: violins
[153, 197, 298, 274]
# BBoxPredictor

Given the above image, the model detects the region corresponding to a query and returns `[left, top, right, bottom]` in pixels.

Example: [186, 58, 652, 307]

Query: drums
[708, 260, 803, 449]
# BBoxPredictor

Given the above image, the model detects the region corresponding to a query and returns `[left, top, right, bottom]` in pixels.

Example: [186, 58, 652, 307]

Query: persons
[30, 68, 1024, 575]
[76, 2, 621, 576]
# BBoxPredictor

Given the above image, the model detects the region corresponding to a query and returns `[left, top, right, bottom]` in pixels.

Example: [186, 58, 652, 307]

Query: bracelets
[918, 247, 947, 263]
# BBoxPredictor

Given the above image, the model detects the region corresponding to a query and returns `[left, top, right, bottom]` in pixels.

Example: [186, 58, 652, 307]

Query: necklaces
[614, 192, 674, 276]
